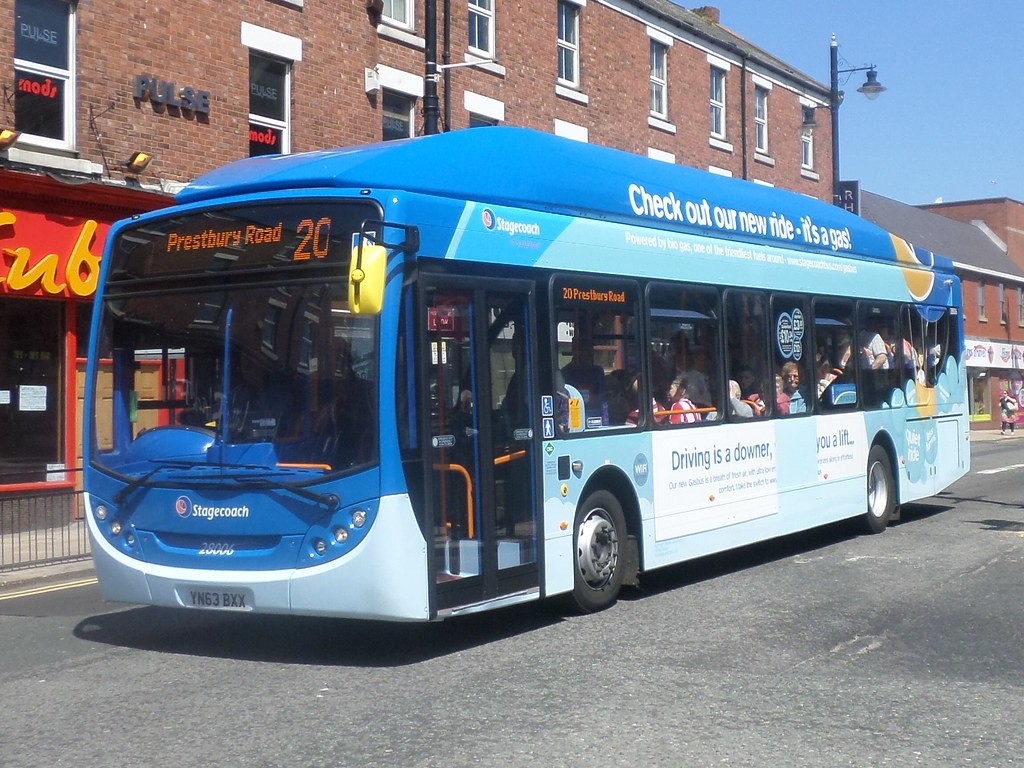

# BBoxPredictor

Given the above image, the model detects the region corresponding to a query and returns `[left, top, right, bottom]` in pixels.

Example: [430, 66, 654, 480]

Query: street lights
[830, 32, 888, 206]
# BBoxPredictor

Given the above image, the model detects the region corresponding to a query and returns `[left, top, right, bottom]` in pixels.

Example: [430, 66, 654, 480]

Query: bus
[78, 124, 973, 632]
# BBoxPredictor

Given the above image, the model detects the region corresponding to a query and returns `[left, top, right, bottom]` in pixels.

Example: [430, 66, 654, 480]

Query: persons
[177, 307, 945, 542]
[998, 390, 1017, 436]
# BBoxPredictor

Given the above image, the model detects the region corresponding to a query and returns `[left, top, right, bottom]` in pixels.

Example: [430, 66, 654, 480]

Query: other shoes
[1011, 432, 1014, 435]
[1001, 431, 1005, 435]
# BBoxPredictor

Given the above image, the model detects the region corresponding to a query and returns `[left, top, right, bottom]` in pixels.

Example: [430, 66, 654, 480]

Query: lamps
[0, 127, 23, 152]
[117, 151, 153, 172]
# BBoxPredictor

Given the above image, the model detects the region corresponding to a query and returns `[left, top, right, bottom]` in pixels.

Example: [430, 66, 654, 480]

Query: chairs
[564, 383, 585, 434]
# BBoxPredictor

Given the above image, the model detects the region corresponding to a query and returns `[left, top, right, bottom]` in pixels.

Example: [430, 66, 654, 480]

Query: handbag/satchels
[1006, 397, 1019, 412]
